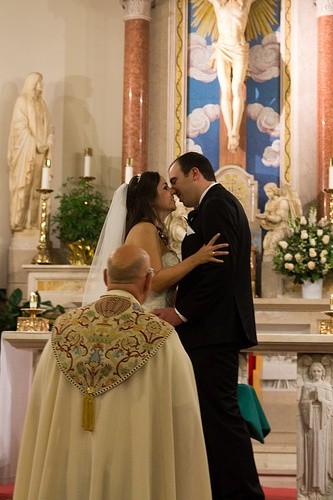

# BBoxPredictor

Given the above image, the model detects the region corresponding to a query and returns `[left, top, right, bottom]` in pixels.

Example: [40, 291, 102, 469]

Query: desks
[0, 331, 333, 486]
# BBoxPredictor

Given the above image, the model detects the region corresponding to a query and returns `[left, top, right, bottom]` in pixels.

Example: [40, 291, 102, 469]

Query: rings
[212, 252, 215, 256]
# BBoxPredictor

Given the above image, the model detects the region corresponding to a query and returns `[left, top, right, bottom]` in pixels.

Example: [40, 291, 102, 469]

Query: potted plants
[51, 175, 110, 267]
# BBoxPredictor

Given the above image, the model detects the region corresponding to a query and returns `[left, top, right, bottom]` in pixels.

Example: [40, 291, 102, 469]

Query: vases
[301, 278, 323, 299]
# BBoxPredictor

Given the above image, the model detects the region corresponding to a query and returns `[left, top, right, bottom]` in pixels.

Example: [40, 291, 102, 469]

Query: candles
[30, 291, 37, 308]
[125, 157, 132, 184]
[329, 158, 333, 189]
[84, 148, 92, 176]
[41, 160, 51, 189]
[330, 294, 333, 310]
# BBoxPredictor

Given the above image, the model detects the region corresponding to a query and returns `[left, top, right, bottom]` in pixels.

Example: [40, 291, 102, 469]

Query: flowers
[272, 206, 333, 285]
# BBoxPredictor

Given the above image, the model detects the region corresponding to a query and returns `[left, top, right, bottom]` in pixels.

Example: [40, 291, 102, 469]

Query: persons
[151, 152, 266, 500]
[12, 247, 212, 500]
[82, 172, 229, 312]
[209, 0, 257, 152]
[300, 362, 333, 495]
[255, 183, 302, 250]
[7, 72, 55, 232]
